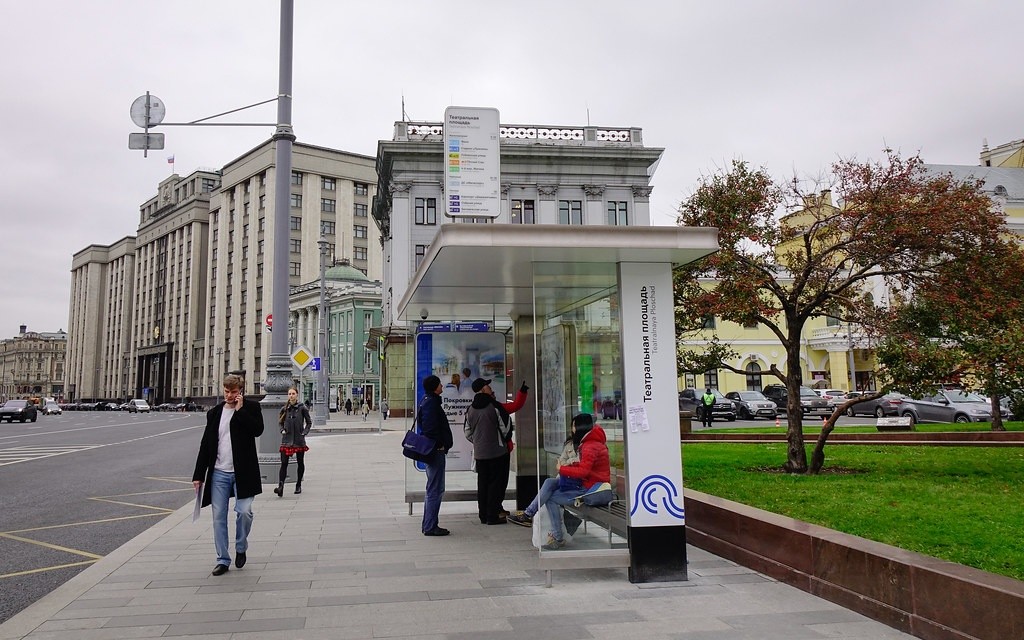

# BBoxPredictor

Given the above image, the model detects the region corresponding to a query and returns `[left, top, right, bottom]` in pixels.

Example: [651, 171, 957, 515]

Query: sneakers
[507, 511, 532, 527]
[541, 536, 566, 551]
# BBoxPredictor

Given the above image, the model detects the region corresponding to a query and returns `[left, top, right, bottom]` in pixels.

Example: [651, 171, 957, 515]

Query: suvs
[678, 387, 737, 423]
[761, 384, 832, 421]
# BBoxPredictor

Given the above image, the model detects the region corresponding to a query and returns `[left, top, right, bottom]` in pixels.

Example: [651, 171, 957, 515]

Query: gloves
[521, 381, 529, 394]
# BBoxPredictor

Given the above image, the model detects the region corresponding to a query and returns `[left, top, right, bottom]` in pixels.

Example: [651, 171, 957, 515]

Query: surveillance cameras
[419, 310, 428, 319]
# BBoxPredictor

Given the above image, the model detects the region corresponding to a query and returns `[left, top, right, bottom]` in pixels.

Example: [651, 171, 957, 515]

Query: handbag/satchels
[402, 430, 436, 465]
[574, 490, 619, 507]
[532, 503, 571, 548]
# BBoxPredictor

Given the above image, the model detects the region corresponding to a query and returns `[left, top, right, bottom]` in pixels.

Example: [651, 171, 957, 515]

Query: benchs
[566, 497, 629, 551]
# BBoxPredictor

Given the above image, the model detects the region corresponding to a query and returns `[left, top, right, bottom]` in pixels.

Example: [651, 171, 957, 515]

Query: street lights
[312, 233, 335, 426]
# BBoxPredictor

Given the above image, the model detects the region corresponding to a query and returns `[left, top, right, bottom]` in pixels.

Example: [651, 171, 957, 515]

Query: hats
[423, 375, 440, 392]
[472, 378, 492, 393]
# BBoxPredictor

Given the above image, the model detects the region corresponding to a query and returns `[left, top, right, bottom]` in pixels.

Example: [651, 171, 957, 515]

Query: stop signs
[266, 313, 273, 327]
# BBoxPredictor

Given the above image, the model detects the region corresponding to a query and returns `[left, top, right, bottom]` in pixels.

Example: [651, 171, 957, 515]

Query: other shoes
[274, 487, 283, 497]
[422, 526, 450, 536]
[481, 517, 507, 525]
[294, 484, 301, 494]
[498, 508, 510, 518]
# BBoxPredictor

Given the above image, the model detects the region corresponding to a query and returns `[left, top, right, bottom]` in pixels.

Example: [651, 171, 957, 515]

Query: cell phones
[443, 106, 502, 217]
[237, 387, 244, 399]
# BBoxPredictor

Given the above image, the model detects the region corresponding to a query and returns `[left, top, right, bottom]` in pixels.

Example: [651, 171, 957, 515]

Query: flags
[168, 156, 174, 163]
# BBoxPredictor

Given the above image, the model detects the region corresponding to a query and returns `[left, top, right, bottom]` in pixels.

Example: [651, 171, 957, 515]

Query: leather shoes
[212, 563, 227, 575]
[235, 550, 246, 568]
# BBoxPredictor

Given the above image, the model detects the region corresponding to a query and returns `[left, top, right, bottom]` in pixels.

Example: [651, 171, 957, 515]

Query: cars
[725, 390, 778, 421]
[812, 388, 848, 415]
[844, 381, 1024, 425]
[35, 396, 204, 417]
[0, 399, 37, 423]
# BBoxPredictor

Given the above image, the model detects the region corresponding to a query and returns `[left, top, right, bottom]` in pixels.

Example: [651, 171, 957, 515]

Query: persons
[701, 389, 716, 428]
[192, 374, 264, 575]
[508, 413, 610, 551]
[305, 397, 389, 422]
[272, 387, 312, 497]
[416, 375, 529, 536]
[449, 368, 474, 392]
